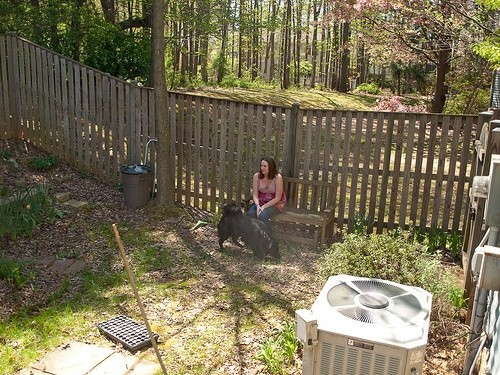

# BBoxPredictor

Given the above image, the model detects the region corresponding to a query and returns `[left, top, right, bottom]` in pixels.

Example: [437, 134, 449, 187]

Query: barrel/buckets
[120, 164, 152, 208]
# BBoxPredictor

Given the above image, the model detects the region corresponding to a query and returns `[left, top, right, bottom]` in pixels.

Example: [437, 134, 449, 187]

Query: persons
[246, 156, 287, 235]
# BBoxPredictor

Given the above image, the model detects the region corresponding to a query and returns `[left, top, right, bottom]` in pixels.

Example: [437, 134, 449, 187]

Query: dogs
[217, 203, 281, 259]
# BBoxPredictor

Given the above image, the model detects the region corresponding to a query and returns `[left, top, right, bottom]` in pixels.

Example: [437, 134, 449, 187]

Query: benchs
[241, 175, 339, 253]
